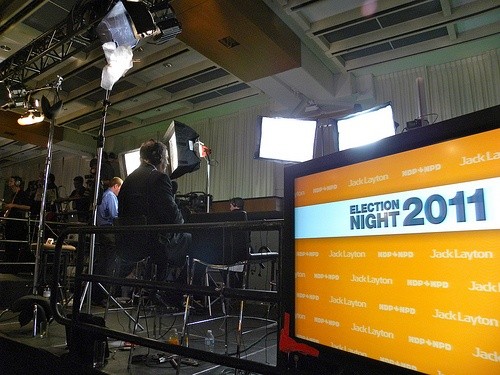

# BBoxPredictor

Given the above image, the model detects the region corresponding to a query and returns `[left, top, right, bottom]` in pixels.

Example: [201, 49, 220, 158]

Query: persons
[0, 173, 91, 259]
[94, 139, 248, 314]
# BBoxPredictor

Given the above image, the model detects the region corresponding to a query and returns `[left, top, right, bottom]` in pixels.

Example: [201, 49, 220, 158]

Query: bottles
[169, 329, 179, 345]
[43, 285, 51, 302]
[204, 329, 215, 354]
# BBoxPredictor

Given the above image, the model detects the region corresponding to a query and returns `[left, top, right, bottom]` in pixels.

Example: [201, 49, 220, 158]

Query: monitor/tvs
[283, 104, 500, 375]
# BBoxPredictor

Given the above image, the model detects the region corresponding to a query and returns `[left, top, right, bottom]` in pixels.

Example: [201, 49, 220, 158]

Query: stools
[31, 243, 76, 305]
[104, 210, 279, 375]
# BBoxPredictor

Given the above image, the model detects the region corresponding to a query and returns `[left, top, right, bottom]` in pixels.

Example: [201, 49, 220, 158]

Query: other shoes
[125, 272, 142, 280]
[101, 299, 116, 307]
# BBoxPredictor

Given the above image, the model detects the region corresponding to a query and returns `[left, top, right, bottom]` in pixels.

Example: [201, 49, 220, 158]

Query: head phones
[15, 177, 21, 187]
[151, 143, 162, 165]
